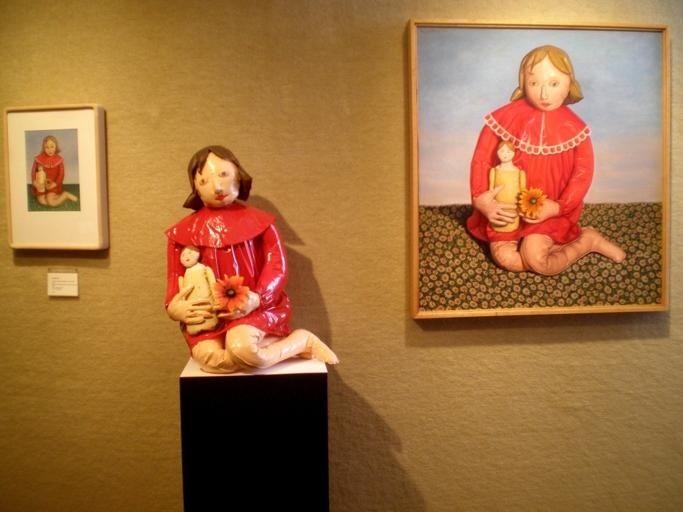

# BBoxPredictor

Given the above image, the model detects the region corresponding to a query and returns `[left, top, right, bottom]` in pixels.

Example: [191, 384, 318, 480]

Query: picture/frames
[3, 103, 109, 255]
[406, 18, 670, 321]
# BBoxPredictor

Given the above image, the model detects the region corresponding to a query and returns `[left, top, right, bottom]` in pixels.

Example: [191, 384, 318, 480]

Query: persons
[489, 141, 526, 233]
[29, 136, 77, 208]
[164, 145, 341, 374]
[463, 43, 627, 276]
[35, 166, 46, 193]
[175, 246, 219, 337]
